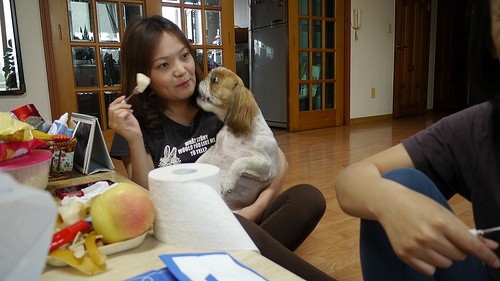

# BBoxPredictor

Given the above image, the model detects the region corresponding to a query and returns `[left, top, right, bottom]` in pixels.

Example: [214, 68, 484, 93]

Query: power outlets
[371, 88, 376, 98]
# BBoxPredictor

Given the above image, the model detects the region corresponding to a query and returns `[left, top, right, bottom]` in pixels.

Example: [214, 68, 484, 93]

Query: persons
[334, 0, 500, 281]
[108, 14, 337, 281]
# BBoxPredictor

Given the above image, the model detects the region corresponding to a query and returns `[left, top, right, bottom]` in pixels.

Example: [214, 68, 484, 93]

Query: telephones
[352, 7, 361, 41]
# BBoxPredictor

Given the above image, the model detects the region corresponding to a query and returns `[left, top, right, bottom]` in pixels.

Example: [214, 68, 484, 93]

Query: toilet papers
[149, 163, 258, 247]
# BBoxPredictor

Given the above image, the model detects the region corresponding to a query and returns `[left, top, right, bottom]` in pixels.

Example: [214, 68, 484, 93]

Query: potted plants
[3, 39, 19, 89]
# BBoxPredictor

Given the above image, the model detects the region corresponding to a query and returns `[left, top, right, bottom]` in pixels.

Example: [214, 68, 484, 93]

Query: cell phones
[55, 180, 115, 199]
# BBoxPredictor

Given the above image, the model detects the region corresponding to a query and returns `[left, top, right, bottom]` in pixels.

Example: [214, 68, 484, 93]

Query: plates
[46, 229, 148, 266]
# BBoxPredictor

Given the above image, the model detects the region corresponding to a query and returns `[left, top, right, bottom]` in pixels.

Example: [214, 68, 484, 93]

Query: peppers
[49, 219, 90, 253]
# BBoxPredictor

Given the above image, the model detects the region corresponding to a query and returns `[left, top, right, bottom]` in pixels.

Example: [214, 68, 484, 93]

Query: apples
[90, 182, 155, 244]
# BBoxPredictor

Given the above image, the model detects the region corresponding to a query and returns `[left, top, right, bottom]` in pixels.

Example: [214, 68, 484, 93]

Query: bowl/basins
[0, 149, 52, 191]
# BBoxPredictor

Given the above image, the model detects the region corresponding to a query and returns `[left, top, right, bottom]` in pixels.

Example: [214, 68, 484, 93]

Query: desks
[40, 167, 307, 281]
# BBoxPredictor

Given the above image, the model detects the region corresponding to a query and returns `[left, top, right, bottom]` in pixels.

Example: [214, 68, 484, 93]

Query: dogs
[194, 66, 280, 211]
